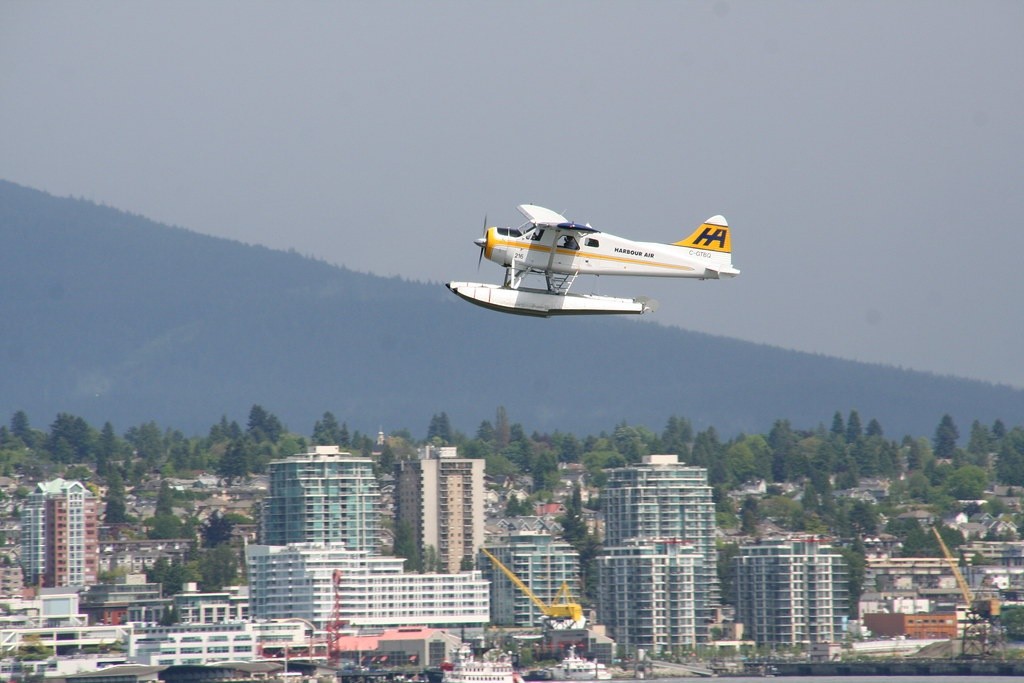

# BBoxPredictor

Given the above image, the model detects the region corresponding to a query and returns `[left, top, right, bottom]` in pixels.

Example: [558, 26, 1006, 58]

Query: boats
[538, 645, 612, 680]
[439, 643, 525, 683]
[0, 655, 66, 683]
[712, 658, 780, 677]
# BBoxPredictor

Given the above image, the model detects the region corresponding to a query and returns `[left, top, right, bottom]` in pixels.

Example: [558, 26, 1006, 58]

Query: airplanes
[444, 204, 740, 318]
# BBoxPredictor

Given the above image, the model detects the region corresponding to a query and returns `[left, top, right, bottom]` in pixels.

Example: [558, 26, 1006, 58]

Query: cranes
[480, 548, 583, 629]
[924, 523, 1006, 654]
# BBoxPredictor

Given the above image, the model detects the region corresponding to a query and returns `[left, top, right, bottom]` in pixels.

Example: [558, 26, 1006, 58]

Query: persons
[564, 236, 577, 249]
